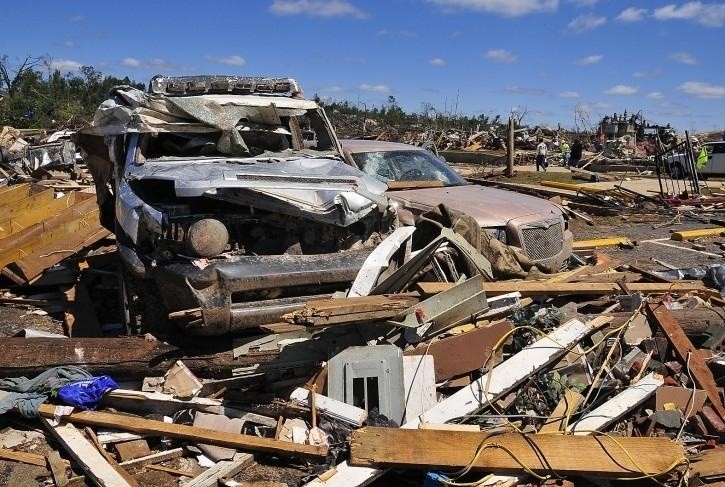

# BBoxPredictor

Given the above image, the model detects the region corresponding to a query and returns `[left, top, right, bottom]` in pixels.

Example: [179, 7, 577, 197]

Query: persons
[692, 138, 703, 158]
[536, 138, 583, 171]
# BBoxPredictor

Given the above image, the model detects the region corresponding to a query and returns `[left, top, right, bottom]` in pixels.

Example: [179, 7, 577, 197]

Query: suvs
[669, 140, 724, 180]
[89, 70, 404, 337]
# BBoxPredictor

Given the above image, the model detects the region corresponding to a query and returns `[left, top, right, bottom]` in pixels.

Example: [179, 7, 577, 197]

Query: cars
[340, 137, 575, 266]
